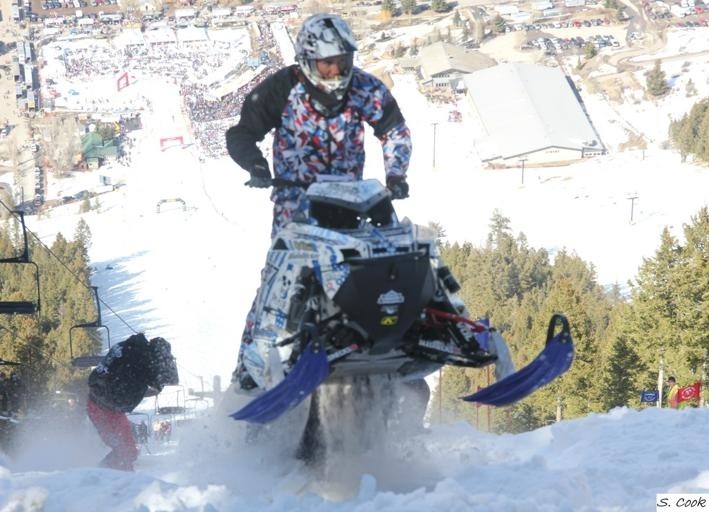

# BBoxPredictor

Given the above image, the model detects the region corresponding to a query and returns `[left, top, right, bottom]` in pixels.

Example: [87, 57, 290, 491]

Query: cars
[21, 1, 124, 27]
[645, 4, 709, 30]
[504, 13, 622, 55]
[1, 129, 9, 136]
[31, 143, 45, 206]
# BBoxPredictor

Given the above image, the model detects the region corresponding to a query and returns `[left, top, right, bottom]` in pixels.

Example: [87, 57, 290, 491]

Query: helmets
[294, 12, 358, 101]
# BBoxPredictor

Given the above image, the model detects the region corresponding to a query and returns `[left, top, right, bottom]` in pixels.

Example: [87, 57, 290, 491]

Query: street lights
[626, 196, 638, 221]
[432, 123, 440, 168]
[520, 159, 528, 184]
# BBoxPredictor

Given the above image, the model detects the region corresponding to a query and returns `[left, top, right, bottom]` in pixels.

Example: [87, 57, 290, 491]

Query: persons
[87, 334, 180, 472]
[133, 419, 171, 444]
[0, 369, 79, 423]
[224, 11, 497, 401]
[665, 376, 686, 409]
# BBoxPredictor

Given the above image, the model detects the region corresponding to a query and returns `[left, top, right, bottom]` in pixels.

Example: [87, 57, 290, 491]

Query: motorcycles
[221, 175, 575, 429]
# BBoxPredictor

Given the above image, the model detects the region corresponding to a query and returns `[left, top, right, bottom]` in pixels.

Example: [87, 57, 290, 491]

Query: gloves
[248, 157, 273, 190]
[387, 177, 409, 200]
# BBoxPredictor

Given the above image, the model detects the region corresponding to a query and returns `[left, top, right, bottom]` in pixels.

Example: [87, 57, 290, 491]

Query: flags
[677, 383, 700, 402]
[640, 389, 659, 402]
